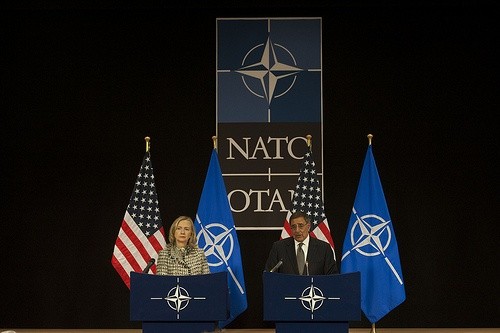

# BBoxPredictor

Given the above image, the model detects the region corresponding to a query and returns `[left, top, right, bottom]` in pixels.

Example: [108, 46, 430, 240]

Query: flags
[111, 142, 167, 290]
[193, 147, 247, 330]
[340, 143, 405, 323]
[280, 145, 336, 261]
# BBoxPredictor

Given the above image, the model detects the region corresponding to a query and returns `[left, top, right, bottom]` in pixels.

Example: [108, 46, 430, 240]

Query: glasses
[289, 223, 308, 229]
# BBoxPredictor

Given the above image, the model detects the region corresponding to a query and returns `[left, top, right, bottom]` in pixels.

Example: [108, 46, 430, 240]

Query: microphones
[143, 258, 155, 273]
[270, 256, 287, 273]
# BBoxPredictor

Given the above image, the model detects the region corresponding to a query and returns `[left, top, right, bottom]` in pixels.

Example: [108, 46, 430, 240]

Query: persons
[156, 216, 211, 275]
[265, 211, 336, 274]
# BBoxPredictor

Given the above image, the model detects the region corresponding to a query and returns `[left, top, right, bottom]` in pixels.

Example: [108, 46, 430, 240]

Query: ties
[296, 243, 305, 275]
